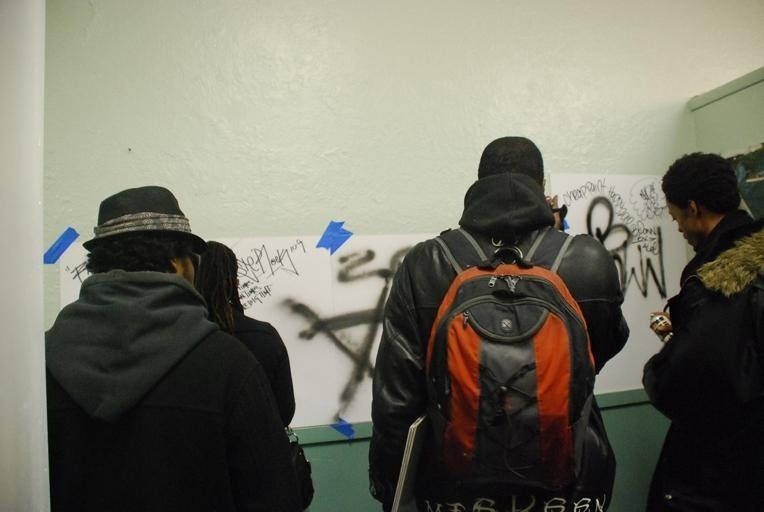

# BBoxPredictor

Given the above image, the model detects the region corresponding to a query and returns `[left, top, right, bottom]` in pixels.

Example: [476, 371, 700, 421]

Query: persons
[44, 185, 317, 511]
[641, 151, 763, 512]
[195, 240, 297, 429]
[368, 136, 630, 512]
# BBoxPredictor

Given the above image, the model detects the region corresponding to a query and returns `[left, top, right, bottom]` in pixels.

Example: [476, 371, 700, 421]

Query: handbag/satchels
[279, 429, 316, 512]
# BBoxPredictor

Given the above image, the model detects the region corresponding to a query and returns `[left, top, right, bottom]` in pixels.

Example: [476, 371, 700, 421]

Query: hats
[80, 184, 206, 252]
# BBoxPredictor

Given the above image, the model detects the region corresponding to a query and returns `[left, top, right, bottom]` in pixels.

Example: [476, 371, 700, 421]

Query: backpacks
[423, 228, 597, 512]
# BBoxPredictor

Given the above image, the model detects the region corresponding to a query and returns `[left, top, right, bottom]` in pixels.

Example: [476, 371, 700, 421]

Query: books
[389, 415, 427, 511]
